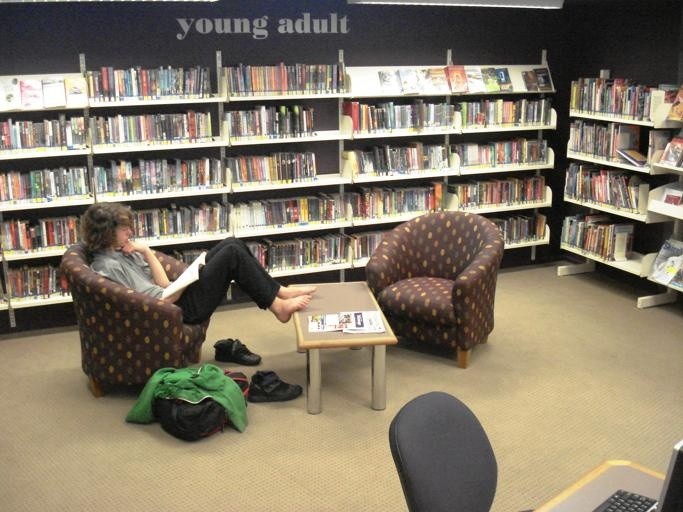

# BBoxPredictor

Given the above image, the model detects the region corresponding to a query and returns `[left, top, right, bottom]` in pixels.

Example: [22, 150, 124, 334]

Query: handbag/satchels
[155, 370, 249, 441]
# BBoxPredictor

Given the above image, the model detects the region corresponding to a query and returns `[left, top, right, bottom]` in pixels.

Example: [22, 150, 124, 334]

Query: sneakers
[248, 370, 302, 401]
[214, 338, 260, 365]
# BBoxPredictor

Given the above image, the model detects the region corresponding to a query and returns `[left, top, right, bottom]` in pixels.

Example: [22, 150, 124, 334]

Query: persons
[78, 200, 317, 323]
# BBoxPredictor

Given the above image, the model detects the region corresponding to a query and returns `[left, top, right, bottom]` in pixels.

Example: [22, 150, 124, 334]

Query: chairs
[388, 390, 497, 511]
[59, 242, 209, 399]
[364, 211, 504, 368]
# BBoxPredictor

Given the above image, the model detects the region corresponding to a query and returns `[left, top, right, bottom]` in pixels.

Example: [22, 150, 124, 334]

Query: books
[224, 66, 341, 272]
[342, 65, 551, 263]
[162, 251, 206, 299]
[559, 76, 681, 290]
[0, 75, 88, 300]
[89, 66, 223, 262]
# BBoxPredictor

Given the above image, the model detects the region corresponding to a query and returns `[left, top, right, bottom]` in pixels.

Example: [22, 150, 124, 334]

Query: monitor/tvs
[654, 441, 683, 512]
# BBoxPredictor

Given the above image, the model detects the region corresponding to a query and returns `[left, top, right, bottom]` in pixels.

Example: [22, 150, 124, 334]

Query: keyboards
[591, 488, 656, 512]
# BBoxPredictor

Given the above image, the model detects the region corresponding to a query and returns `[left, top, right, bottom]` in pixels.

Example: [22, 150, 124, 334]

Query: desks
[285, 279, 398, 414]
